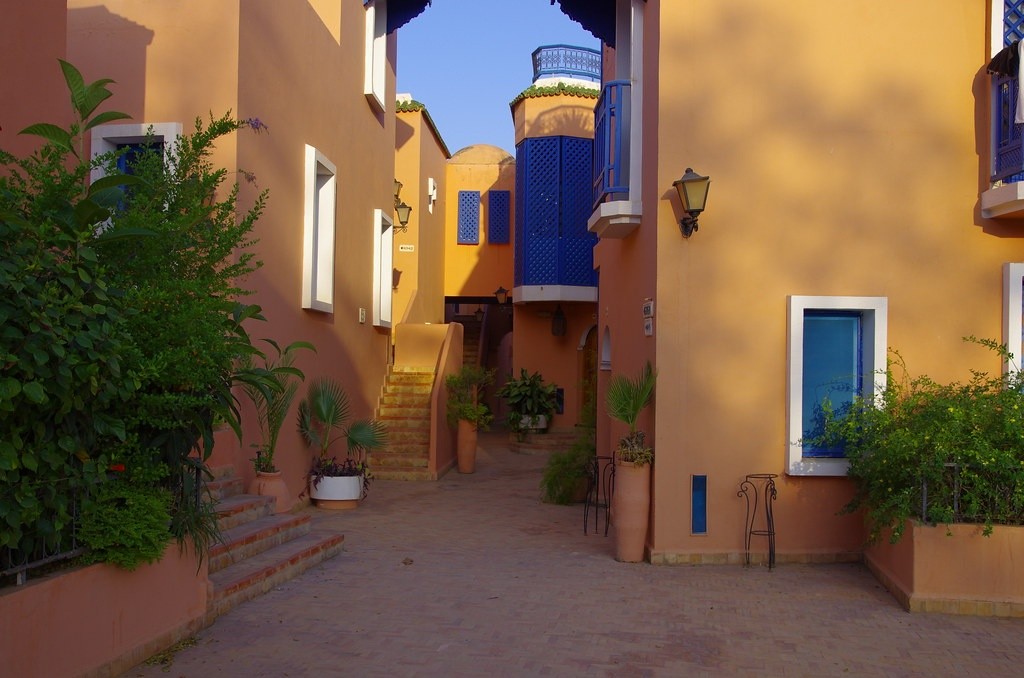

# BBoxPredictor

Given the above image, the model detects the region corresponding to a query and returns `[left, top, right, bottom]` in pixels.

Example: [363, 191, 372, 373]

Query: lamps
[392, 267, 403, 289]
[495, 286, 513, 313]
[393, 202, 412, 235]
[551, 305, 567, 336]
[394, 179, 403, 198]
[474, 304, 483, 321]
[674, 166, 710, 239]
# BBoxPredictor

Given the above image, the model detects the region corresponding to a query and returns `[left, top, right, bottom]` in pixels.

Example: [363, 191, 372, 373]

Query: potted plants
[241, 340, 315, 514]
[445, 367, 495, 477]
[606, 362, 654, 562]
[297, 380, 385, 502]
[495, 368, 557, 433]
